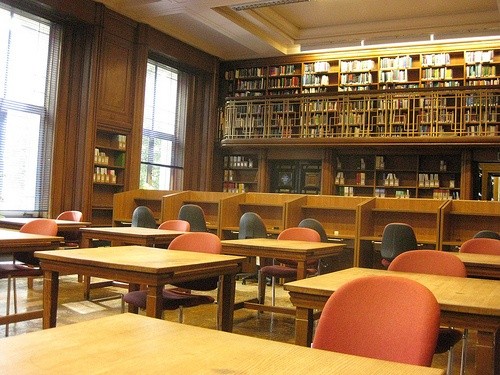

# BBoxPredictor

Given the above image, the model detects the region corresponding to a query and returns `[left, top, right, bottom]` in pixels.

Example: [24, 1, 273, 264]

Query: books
[92, 132, 127, 183]
[218, 49, 500, 201]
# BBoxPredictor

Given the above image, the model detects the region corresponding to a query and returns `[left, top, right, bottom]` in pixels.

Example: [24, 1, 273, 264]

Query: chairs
[56, 210, 84, 250]
[179, 204, 207, 232]
[123, 231, 224, 323]
[130, 205, 156, 228]
[311, 273, 442, 370]
[376, 221, 417, 271]
[386, 249, 469, 375]
[258, 227, 324, 307]
[236, 211, 273, 286]
[0, 218, 58, 336]
[472, 230, 500, 239]
[299, 217, 330, 273]
[153, 220, 191, 248]
[458, 238, 500, 255]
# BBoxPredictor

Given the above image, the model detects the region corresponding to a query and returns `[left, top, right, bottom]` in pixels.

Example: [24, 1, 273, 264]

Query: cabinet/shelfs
[211, 39, 500, 200]
[88, 122, 132, 225]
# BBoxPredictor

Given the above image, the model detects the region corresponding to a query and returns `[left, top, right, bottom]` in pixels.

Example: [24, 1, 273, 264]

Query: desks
[0, 188, 500, 375]
